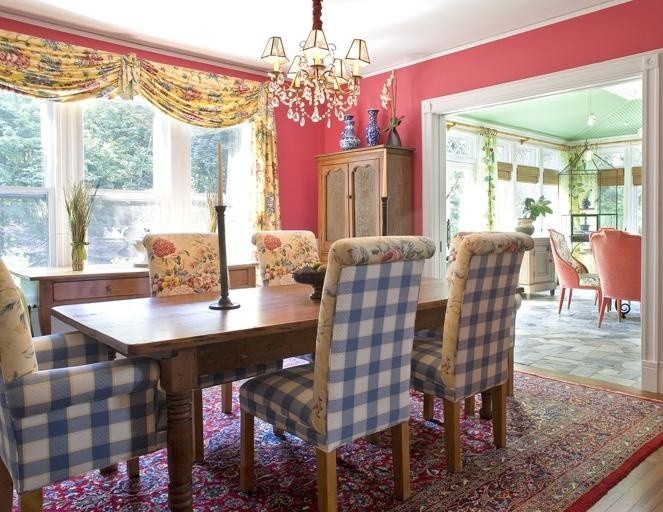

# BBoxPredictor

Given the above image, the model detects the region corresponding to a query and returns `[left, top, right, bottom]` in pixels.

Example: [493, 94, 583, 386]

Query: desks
[51, 278, 482, 512]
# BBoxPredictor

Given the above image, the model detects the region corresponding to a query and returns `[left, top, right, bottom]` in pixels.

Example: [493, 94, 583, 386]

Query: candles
[217, 143, 224, 206]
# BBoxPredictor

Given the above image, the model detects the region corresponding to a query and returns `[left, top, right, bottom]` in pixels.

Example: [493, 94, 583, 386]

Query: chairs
[143, 233, 283, 466]
[590, 228, 641, 328]
[232, 236, 437, 512]
[0, 259, 167, 512]
[413, 233, 534, 472]
[252, 230, 317, 288]
[547, 230, 600, 314]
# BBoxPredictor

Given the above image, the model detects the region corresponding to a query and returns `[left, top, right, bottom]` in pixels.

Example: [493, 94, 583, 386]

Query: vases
[339, 115, 361, 149]
[580, 225, 589, 231]
[364, 110, 380, 146]
[70, 241, 87, 271]
[385, 127, 402, 146]
[581, 199, 591, 209]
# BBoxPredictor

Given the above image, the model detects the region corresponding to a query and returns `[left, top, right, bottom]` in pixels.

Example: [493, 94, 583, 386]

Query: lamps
[261, 0, 370, 128]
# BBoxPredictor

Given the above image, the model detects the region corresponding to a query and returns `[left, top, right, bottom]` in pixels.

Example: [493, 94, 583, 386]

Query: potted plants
[514, 195, 554, 236]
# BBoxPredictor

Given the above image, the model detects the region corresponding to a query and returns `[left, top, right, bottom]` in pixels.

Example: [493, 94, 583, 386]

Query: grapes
[293, 264, 316, 274]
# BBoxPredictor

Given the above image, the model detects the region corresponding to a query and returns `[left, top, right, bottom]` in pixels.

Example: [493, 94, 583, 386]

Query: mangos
[317, 264, 327, 273]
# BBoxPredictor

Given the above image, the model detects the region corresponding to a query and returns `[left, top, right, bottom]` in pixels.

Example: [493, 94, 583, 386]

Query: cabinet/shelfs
[9, 262, 259, 332]
[314, 144, 416, 264]
[517, 238, 558, 300]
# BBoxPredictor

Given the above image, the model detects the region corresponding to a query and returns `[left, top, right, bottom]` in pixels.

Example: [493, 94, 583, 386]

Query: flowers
[380, 70, 405, 127]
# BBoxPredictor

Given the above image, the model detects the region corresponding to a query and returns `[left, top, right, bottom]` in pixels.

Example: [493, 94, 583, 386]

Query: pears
[313, 263, 322, 270]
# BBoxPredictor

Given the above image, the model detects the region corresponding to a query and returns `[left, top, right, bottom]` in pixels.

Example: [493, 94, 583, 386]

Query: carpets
[0, 357, 663, 512]
